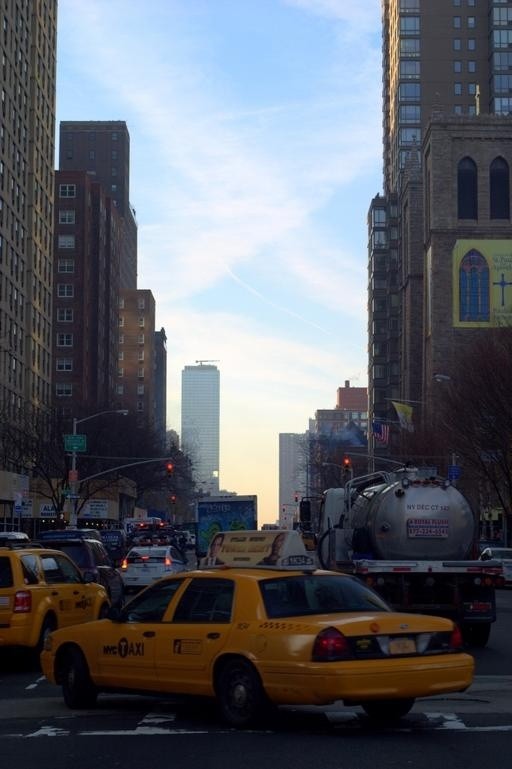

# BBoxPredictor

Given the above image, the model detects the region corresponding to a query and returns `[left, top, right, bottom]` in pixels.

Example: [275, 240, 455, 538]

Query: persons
[201, 533, 227, 566]
[256, 530, 285, 567]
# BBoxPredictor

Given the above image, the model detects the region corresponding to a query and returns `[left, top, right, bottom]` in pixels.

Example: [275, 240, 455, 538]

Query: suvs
[0, 543, 114, 669]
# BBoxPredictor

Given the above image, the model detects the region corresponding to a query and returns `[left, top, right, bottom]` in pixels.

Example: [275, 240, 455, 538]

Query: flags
[390, 400, 414, 435]
[372, 420, 389, 446]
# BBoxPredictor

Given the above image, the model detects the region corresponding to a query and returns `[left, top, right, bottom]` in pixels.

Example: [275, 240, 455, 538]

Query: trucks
[193, 493, 259, 570]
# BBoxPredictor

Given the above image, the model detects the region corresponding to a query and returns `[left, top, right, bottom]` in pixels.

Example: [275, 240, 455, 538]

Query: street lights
[66, 408, 129, 527]
[432, 374, 467, 384]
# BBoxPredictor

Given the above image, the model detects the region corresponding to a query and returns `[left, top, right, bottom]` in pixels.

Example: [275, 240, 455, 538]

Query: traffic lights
[294, 494, 300, 503]
[167, 463, 173, 478]
[342, 454, 351, 484]
[171, 494, 176, 504]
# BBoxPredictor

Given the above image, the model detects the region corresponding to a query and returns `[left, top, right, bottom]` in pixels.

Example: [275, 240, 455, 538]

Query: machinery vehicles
[299, 464, 505, 655]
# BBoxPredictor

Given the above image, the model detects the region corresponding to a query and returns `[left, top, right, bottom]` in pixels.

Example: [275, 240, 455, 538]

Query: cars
[40, 529, 477, 731]
[476, 545, 512, 588]
[1, 511, 194, 607]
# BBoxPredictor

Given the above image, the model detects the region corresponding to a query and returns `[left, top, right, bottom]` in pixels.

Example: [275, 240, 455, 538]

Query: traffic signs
[63, 494, 81, 499]
[62, 434, 87, 451]
